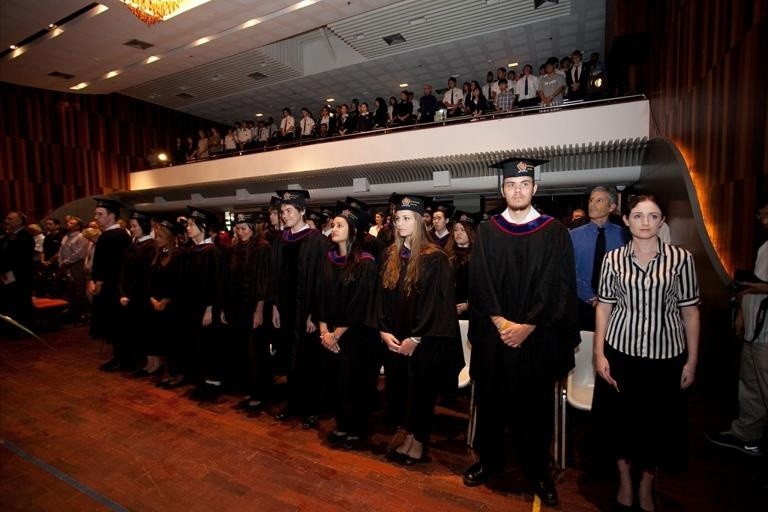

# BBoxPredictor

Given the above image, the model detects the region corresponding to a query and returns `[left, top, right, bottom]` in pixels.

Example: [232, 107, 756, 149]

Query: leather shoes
[302, 413, 321, 430]
[525, 463, 560, 506]
[275, 407, 292, 419]
[462, 455, 504, 486]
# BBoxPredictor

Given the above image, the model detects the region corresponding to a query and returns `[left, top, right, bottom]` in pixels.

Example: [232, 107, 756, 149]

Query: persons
[173, 136, 187, 163]
[189, 245, 231, 402]
[270, 189, 327, 434]
[369, 211, 382, 237]
[349, 99, 360, 131]
[373, 97, 388, 127]
[314, 201, 376, 449]
[418, 84, 438, 123]
[242, 120, 251, 142]
[279, 107, 295, 136]
[560, 57, 572, 72]
[317, 105, 334, 137]
[209, 127, 221, 152]
[494, 78, 514, 118]
[463, 157, 581, 508]
[388, 96, 398, 127]
[394, 90, 412, 125]
[198, 130, 209, 159]
[537, 64, 546, 78]
[359, 102, 372, 131]
[87, 197, 132, 372]
[258, 120, 269, 141]
[264, 196, 284, 241]
[465, 80, 481, 114]
[58, 216, 88, 326]
[105, 209, 158, 372]
[586, 52, 602, 71]
[133, 220, 179, 377]
[321, 209, 337, 251]
[376, 193, 465, 466]
[507, 70, 515, 81]
[593, 194, 700, 510]
[462, 82, 470, 115]
[27, 223, 44, 263]
[423, 203, 432, 229]
[156, 205, 221, 390]
[444, 212, 481, 261]
[566, 50, 589, 100]
[568, 186, 630, 331]
[41, 218, 63, 298]
[482, 71, 496, 110]
[571, 210, 585, 221]
[492, 67, 513, 102]
[516, 63, 541, 115]
[470, 87, 487, 122]
[308, 211, 322, 232]
[707, 196, 768, 457]
[538, 61, 566, 112]
[299, 107, 315, 138]
[377, 193, 399, 254]
[409, 91, 420, 120]
[0, 211, 36, 339]
[222, 210, 271, 413]
[233, 121, 245, 150]
[335, 106, 340, 118]
[443, 77, 463, 116]
[336, 104, 352, 134]
[186, 135, 197, 160]
[173, 222, 189, 246]
[225, 130, 237, 151]
[548, 57, 564, 76]
[248, 120, 258, 141]
[431, 201, 454, 246]
[117, 218, 130, 234]
[266, 117, 277, 137]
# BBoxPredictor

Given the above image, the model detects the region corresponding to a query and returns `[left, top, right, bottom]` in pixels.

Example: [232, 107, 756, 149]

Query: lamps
[120, 0, 211, 27]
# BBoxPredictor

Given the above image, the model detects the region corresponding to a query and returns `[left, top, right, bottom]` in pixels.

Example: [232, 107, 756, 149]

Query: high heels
[401, 437, 431, 465]
[390, 428, 414, 461]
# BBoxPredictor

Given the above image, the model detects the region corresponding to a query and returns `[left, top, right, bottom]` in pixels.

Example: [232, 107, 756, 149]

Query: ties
[260, 128, 263, 139]
[284, 117, 288, 131]
[303, 119, 307, 133]
[525, 76, 528, 95]
[451, 90, 454, 105]
[591, 229, 607, 295]
[574, 65, 579, 83]
[489, 84, 491, 98]
[269, 126, 272, 138]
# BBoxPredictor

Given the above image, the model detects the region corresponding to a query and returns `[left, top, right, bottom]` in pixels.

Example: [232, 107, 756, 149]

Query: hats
[386, 192, 434, 216]
[305, 208, 328, 225]
[274, 188, 311, 203]
[344, 195, 372, 212]
[92, 197, 126, 216]
[185, 204, 216, 222]
[160, 218, 184, 236]
[332, 198, 372, 224]
[487, 156, 551, 178]
[232, 210, 261, 224]
[452, 208, 477, 225]
[128, 208, 154, 221]
[429, 200, 456, 216]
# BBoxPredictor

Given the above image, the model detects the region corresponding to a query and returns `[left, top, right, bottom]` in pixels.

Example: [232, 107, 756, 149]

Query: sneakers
[156, 375, 175, 387]
[327, 424, 349, 443]
[165, 377, 187, 389]
[707, 428, 762, 458]
[246, 399, 265, 411]
[239, 396, 253, 409]
[339, 432, 371, 449]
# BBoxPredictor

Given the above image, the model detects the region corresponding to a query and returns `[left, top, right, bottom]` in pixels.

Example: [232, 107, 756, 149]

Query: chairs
[560, 331, 597, 470]
[457, 320, 473, 447]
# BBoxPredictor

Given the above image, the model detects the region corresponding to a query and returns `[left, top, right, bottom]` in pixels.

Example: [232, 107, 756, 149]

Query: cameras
[729, 266, 764, 295]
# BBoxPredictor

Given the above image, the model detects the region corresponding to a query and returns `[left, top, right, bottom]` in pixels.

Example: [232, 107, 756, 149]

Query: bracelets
[411, 337, 421, 344]
